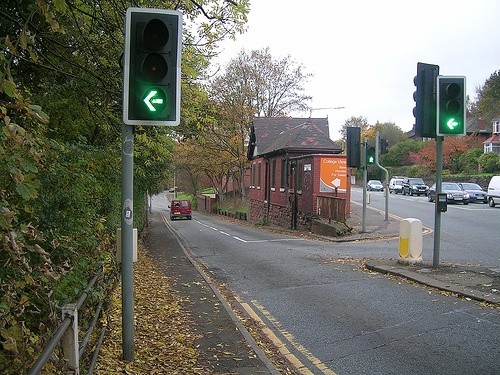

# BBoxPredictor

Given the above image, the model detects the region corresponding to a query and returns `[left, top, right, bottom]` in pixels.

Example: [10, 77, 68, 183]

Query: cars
[168, 188, 174, 193]
[367, 179, 385, 192]
[427, 181, 471, 205]
[457, 183, 488, 204]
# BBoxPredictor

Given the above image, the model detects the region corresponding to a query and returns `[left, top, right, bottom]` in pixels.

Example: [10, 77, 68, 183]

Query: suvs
[401, 177, 430, 196]
[389, 177, 405, 194]
[168, 199, 192, 220]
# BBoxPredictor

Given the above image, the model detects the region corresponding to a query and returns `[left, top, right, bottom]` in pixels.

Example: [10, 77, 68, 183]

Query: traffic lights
[412, 62, 437, 138]
[381, 139, 390, 154]
[436, 76, 466, 136]
[366, 143, 376, 164]
[122, 7, 182, 127]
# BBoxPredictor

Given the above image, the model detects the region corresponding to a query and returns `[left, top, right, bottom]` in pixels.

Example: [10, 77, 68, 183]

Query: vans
[487, 175, 500, 207]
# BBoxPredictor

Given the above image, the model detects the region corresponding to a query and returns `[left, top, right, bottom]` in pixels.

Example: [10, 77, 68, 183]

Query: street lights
[310, 106, 345, 118]
